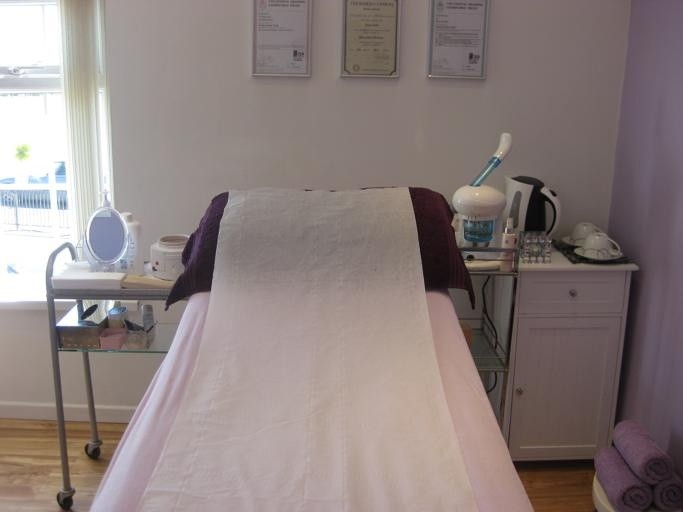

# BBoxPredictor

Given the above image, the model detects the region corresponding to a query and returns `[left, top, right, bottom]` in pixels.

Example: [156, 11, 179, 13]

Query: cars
[0, 162, 67, 209]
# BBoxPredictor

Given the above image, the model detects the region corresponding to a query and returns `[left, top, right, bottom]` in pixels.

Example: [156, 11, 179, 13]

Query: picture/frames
[249, 1, 491, 82]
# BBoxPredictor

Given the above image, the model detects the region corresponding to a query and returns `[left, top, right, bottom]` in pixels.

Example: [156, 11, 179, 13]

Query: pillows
[163, 187, 476, 310]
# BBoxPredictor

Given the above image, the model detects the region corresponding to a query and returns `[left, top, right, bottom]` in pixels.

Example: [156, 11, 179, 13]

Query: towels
[592, 420, 682, 512]
[134, 188, 478, 511]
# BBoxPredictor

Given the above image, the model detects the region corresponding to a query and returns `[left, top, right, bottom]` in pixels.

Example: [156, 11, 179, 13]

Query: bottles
[505, 217, 516, 234]
[111, 211, 145, 277]
[149, 234, 192, 282]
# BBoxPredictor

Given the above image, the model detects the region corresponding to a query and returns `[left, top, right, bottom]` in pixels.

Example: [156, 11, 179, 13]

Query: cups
[570, 222, 621, 258]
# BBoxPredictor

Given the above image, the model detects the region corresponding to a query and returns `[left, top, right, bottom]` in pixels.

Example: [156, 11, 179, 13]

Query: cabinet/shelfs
[454, 245, 520, 431]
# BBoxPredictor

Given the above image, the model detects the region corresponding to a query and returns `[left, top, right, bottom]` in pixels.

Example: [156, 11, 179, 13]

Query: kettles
[501, 175, 562, 237]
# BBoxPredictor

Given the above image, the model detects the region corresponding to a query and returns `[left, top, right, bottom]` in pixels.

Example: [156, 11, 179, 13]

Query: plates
[561, 236, 623, 261]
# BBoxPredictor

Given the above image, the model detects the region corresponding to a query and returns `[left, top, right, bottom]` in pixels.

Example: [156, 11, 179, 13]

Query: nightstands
[492, 242, 638, 466]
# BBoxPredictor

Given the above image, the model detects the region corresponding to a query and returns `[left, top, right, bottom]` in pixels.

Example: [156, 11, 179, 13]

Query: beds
[90, 181, 535, 512]
